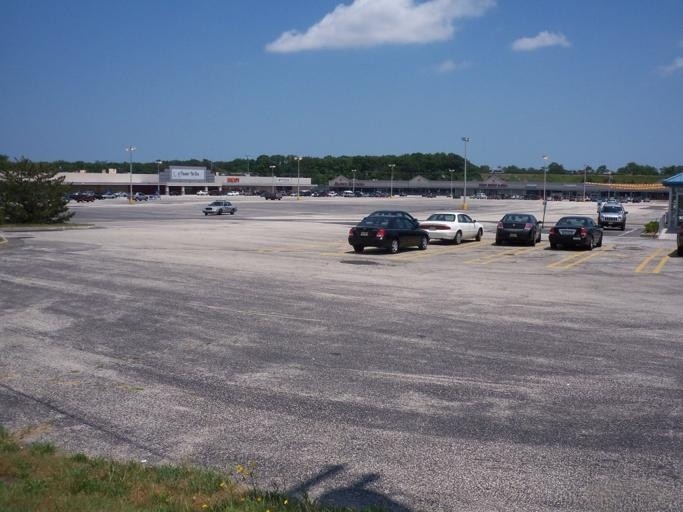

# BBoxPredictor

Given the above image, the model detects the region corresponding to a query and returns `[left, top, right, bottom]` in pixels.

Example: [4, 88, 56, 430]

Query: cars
[63, 189, 240, 203]
[549, 215, 603, 252]
[201, 199, 238, 215]
[494, 213, 544, 246]
[348, 209, 485, 255]
[250, 186, 385, 201]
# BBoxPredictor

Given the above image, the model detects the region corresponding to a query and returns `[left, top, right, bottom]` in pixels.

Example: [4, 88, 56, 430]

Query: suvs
[596, 202, 628, 231]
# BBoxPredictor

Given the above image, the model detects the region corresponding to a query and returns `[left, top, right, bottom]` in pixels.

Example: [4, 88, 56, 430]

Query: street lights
[460, 136, 470, 205]
[448, 169, 455, 196]
[351, 169, 356, 192]
[269, 164, 276, 194]
[388, 163, 395, 197]
[541, 155, 615, 203]
[294, 156, 303, 200]
[155, 159, 163, 195]
[125, 146, 136, 203]
